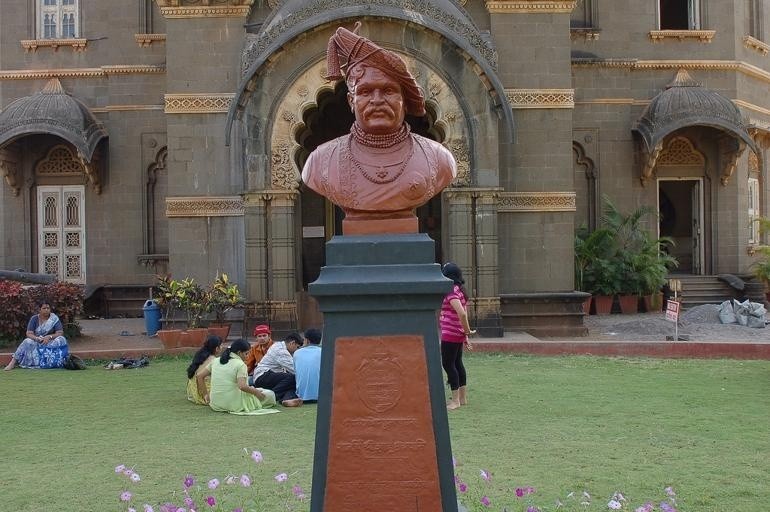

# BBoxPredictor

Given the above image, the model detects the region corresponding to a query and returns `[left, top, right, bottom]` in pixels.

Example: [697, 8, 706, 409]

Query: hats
[441, 262, 465, 285]
[254, 324, 272, 336]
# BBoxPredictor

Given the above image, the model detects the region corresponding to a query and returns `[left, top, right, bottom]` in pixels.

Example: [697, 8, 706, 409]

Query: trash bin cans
[142, 300, 162, 337]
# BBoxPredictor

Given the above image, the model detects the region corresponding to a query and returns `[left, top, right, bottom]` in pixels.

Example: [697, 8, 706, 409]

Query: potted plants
[151, 269, 247, 348]
[575, 193, 680, 313]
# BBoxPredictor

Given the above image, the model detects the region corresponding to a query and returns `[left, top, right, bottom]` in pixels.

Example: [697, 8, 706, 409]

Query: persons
[196, 338, 277, 412]
[291, 327, 323, 403]
[439, 262, 477, 410]
[4, 299, 68, 371]
[185, 335, 223, 406]
[246, 324, 275, 373]
[252, 331, 305, 407]
[297, 24, 459, 207]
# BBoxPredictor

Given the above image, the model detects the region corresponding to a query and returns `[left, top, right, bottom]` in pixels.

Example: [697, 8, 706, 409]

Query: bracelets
[48, 334, 53, 340]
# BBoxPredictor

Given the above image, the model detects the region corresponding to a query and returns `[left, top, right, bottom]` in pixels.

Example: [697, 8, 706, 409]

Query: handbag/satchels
[64, 354, 87, 370]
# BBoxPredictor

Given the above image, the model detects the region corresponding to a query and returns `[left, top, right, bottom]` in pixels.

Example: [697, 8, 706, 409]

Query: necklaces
[347, 120, 415, 186]
